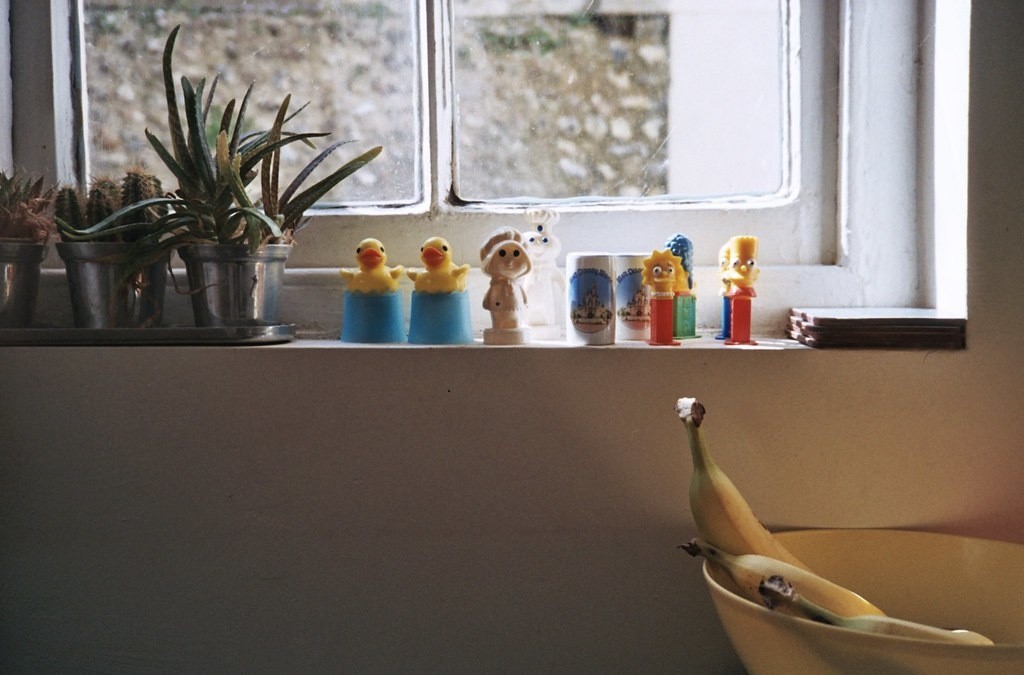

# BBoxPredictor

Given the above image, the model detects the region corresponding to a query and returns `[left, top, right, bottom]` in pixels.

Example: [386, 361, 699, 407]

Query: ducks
[407, 237, 470, 294]
[338, 237, 404, 294]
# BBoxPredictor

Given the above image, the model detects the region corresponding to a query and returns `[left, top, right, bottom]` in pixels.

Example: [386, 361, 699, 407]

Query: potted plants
[0, 166, 60, 329]
[53, 24, 382, 327]
[53, 164, 177, 327]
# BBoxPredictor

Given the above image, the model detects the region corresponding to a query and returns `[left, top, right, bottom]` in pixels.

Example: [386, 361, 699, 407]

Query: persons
[479, 225, 531, 328]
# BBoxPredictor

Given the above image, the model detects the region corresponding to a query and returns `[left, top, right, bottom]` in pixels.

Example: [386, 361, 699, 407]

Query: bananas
[681, 537, 888, 624]
[757, 573, 996, 646]
[674, 398, 809, 568]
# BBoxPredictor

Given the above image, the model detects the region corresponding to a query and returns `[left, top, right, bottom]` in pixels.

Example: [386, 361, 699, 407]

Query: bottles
[615, 254, 653, 340]
[567, 252, 615, 344]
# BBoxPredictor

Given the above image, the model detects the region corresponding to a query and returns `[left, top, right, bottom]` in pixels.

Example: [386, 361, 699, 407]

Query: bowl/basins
[702, 530, 1024, 675]
